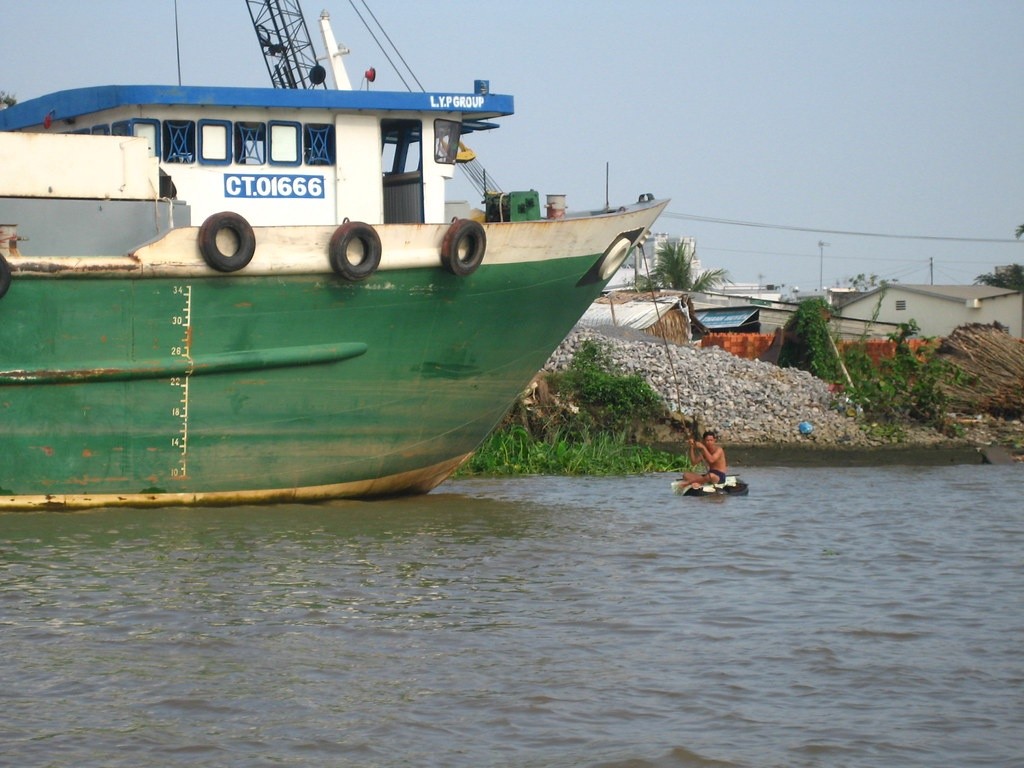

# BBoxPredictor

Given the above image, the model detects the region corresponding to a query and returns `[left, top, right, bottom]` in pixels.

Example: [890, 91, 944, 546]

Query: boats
[0, 0, 674, 510]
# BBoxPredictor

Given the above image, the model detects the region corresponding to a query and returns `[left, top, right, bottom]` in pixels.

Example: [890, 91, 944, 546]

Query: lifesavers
[329, 220, 382, 282]
[198, 210, 257, 272]
[441, 218, 487, 277]
[0, 252, 12, 299]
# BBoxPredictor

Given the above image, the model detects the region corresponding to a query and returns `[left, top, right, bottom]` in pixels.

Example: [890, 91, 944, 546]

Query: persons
[679, 432, 726, 489]
